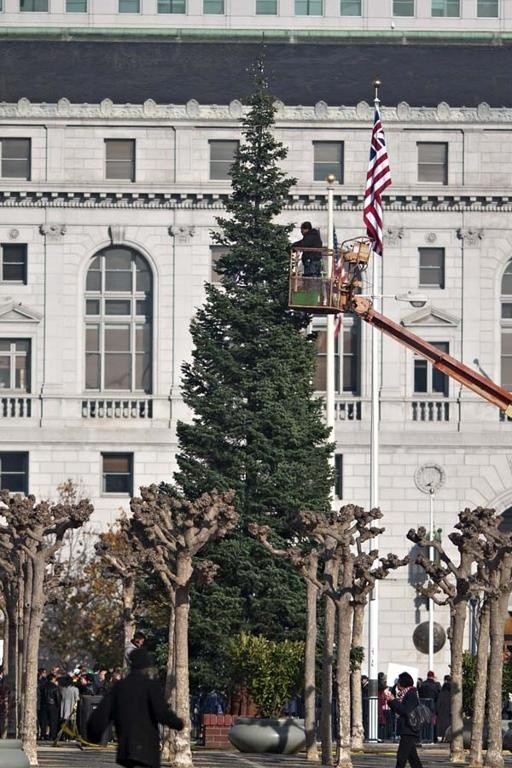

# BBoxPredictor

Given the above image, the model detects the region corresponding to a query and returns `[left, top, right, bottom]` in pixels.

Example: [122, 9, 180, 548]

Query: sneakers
[0, 731, 77, 741]
[377, 734, 448, 744]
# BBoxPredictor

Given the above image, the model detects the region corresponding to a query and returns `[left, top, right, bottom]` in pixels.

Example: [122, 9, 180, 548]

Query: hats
[398, 672, 414, 686]
[125, 647, 157, 668]
[428, 671, 434, 677]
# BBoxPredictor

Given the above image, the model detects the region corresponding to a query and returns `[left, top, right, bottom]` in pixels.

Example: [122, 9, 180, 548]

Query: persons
[0, 664, 127, 745]
[87, 647, 188, 767]
[385, 672, 423, 768]
[132, 639, 159, 676]
[361, 671, 452, 742]
[124, 633, 146, 668]
[288, 220, 323, 293]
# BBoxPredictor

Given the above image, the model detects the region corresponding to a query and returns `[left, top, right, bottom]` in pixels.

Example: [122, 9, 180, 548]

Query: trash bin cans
[79, 695, 109, 747]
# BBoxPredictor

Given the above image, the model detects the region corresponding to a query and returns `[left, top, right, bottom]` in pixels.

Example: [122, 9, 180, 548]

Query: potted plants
[223, 628, 323, 755]
[446, 649, 512, 749]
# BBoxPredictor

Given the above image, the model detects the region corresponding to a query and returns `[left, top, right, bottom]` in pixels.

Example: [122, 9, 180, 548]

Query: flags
[364, 107, 393, 257]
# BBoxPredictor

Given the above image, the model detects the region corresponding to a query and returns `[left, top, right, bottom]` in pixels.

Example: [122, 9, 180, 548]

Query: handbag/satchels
[408, 686, 434, 732]
